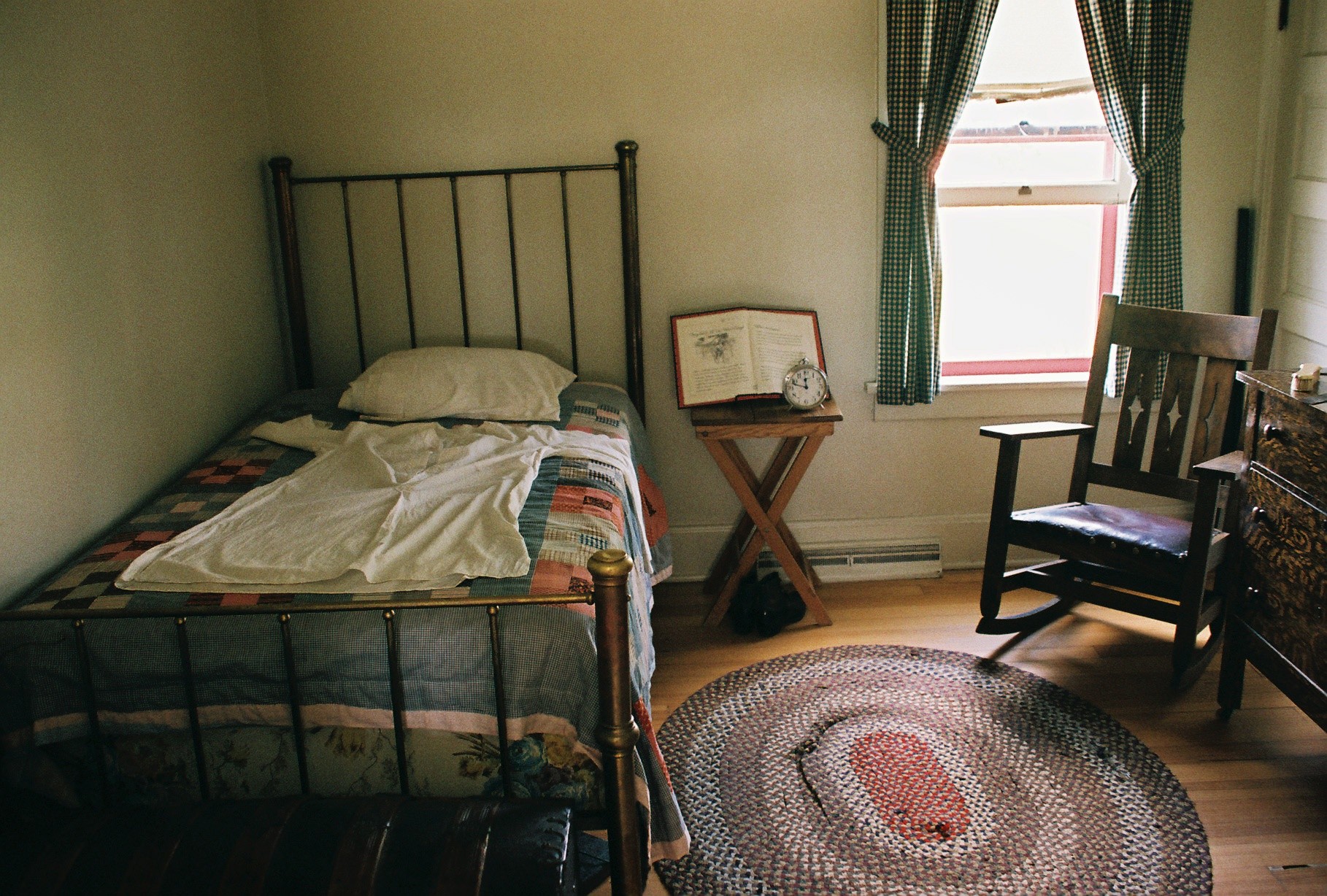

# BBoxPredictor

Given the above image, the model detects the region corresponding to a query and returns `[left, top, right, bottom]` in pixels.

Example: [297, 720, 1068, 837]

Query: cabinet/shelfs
[1217, 370, 1327, 727]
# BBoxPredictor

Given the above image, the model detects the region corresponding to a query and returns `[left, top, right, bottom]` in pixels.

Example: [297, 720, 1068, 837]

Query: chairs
[974, 294, 1280, 687]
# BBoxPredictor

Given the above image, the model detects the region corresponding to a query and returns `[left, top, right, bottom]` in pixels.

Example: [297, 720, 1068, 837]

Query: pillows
[335, 346, 579, 423]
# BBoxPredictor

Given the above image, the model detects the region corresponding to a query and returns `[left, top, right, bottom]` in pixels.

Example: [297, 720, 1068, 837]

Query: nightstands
[689, 397, 844, 629]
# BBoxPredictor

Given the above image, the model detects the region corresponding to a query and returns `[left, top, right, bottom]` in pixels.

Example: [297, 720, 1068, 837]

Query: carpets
[653, 644, 1211, 896]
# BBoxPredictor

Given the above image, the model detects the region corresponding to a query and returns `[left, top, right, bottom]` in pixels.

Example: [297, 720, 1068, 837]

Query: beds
[0, 142, 673, 896]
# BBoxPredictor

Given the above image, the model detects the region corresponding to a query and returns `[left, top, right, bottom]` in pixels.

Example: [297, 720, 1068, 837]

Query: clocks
[782, 358, 828, 413]
